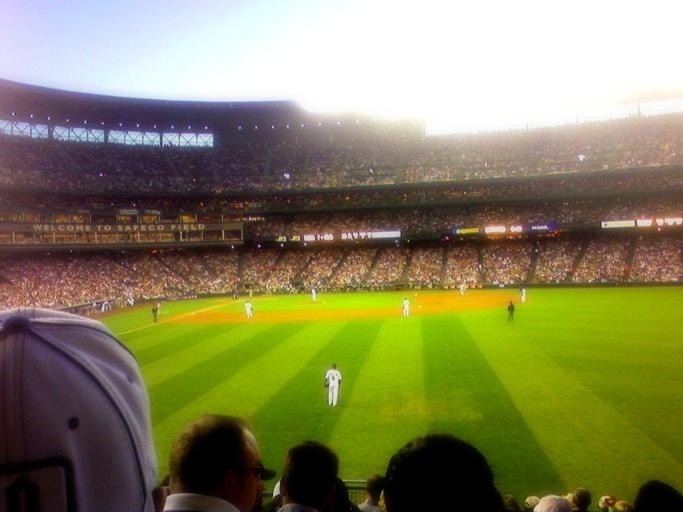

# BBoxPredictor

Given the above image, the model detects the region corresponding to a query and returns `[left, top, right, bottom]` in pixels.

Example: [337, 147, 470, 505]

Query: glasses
[222, 463, 276, 482]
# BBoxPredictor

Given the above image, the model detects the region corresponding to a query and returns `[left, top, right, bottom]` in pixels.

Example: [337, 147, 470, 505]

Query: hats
[0, 308, 159, 511]
